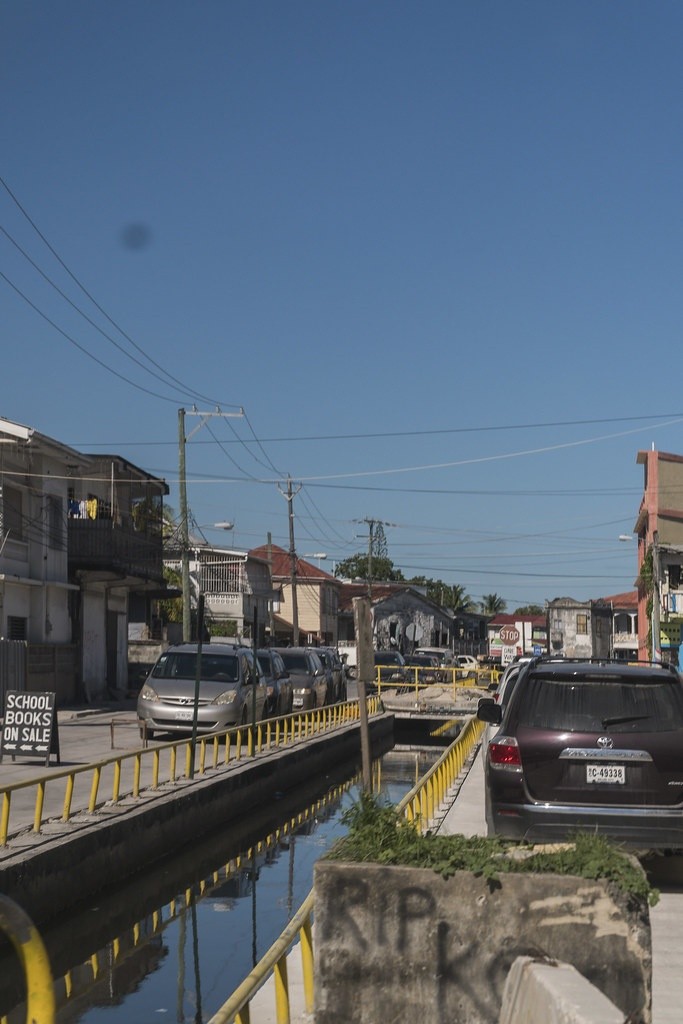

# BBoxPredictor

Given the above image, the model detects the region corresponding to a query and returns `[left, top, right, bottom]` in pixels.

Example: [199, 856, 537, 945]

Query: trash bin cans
[128, 662, 154, 690]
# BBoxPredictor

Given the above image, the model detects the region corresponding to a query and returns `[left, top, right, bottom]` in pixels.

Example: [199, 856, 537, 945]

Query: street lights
[615, 532, 662, 665]
[290, 551, 329, 645]
[182, 520, 235, 643]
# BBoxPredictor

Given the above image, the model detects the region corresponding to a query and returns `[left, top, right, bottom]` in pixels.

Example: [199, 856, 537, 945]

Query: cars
[270, 645, 350, 720]
[136, 640, 269, 746]
[456, 655, 481, 678]
[250, 646, 294, 730]
[373, 649, 448, 696]
[475, 650, 683, 873]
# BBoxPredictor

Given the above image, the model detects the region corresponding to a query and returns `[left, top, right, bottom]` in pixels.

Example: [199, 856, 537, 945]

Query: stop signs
[499, 625, 522, 646]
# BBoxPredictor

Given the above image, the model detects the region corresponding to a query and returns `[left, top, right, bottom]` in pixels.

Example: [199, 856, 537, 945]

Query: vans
[413, 646, 461, 685]
